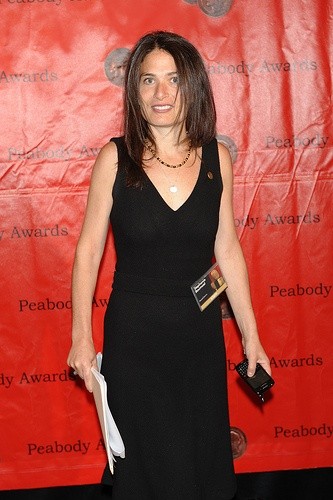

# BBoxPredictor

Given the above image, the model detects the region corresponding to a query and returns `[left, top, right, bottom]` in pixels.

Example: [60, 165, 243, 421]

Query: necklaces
[146, 139, 192, 168]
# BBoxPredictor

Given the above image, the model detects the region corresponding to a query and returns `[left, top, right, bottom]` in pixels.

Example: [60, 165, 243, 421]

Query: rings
[74, 370, 78, 375]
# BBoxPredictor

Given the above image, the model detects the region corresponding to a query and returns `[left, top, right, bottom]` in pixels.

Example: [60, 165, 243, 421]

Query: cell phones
[235, 358, 274, 403]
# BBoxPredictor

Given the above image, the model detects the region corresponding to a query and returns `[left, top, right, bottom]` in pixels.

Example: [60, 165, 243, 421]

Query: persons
[68, 31, 272, 500]
